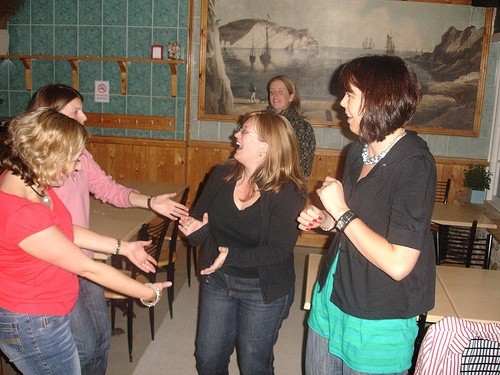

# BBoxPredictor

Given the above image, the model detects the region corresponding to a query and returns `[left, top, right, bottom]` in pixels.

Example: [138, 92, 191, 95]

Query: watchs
[335, 209, 359, 233]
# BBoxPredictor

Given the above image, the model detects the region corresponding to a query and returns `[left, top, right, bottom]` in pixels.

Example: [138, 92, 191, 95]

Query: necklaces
[28, 184, 51, 204]
[361, 130, 405, 166]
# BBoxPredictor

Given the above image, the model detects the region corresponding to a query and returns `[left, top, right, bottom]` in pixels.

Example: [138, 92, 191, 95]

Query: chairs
[428, 179, 492, 269]
[413, 314, 500, 375]
[103, 173, 208, 362]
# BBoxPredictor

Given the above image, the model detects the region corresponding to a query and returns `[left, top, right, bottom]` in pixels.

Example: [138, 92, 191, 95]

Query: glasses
[237, 126, 261, 141]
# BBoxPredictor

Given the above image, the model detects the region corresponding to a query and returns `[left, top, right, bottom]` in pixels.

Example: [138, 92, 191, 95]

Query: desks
[89, 216, 146, 261]
[431, 202, 497, 228]
[435, 267, 500, 323]
[296, 230, 337, 310]
[303, 254, 457, 323]
[90, 179, 189, 224]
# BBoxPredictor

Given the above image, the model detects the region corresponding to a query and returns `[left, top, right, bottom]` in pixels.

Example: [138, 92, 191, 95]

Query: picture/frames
[198, 0, 493, 137]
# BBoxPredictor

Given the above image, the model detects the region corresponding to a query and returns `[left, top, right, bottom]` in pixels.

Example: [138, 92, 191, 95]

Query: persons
[178, 109, 304, 375]
[267, 75, 317, 180]
[0, 85, 190, 374]
[294, 56, 436, 375]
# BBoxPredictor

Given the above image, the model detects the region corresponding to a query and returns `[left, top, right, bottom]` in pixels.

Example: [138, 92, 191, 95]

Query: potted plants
[462, 164, 494, 204]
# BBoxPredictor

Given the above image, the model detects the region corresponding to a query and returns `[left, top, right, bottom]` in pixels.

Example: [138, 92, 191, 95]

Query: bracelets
[113, 238, 121, 256]
[147, 195, 155, 210]
[140, 283, 161, 307]
[320, 218, 337, 232]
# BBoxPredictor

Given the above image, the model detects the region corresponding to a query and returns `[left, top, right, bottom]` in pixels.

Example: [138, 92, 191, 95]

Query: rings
[143, 262, 146, 266]
